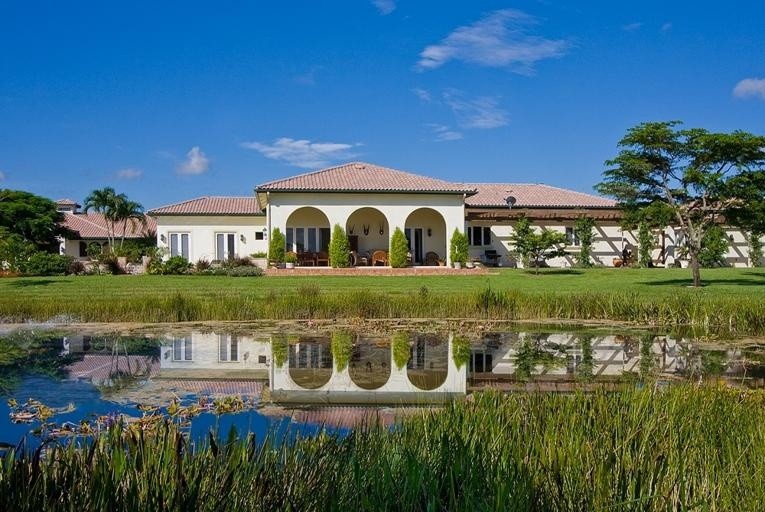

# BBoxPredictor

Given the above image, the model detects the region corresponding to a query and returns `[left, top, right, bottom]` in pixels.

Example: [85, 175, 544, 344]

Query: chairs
[350, 250, 388, 266]
[425, 251, 439, 265]
[299, 250, 329, 266]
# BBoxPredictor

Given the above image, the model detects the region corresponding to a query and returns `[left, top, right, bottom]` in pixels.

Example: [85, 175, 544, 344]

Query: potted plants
[283, 250, 298, 269]
[451, 253, 464, 270]
